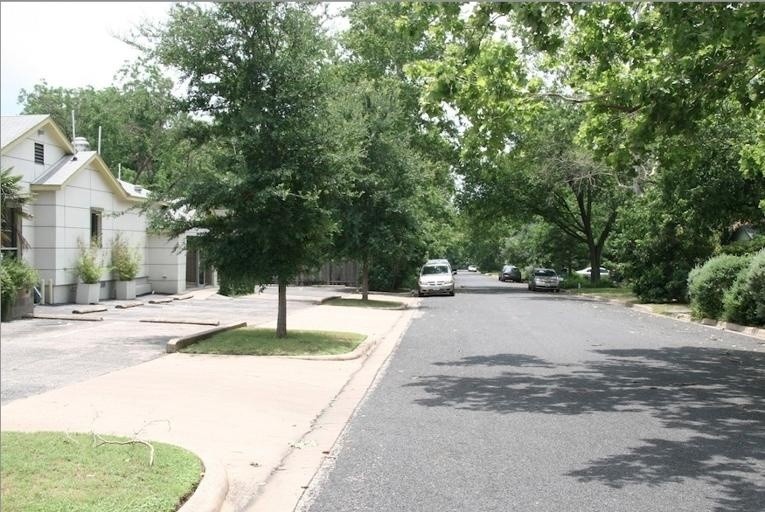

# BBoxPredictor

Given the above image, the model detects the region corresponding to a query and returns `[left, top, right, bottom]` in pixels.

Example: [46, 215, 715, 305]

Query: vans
[497, 264, 522, 282]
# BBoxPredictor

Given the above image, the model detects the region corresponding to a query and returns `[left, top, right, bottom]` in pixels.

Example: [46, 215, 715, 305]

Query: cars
[528, 267, 561, 293]
[452, 265, 457, 275]
[575, 265, 612, 280]
[467, 264, 479, 272]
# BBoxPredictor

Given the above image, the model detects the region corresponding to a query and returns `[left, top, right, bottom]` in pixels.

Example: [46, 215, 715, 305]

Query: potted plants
[0, 249, 40, 324]
[109, 232, 142, 302]
[73, 229, 107, 306]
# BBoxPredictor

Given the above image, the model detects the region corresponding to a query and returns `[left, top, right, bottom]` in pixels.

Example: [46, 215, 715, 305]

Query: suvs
[413, 259, 458, 297]
